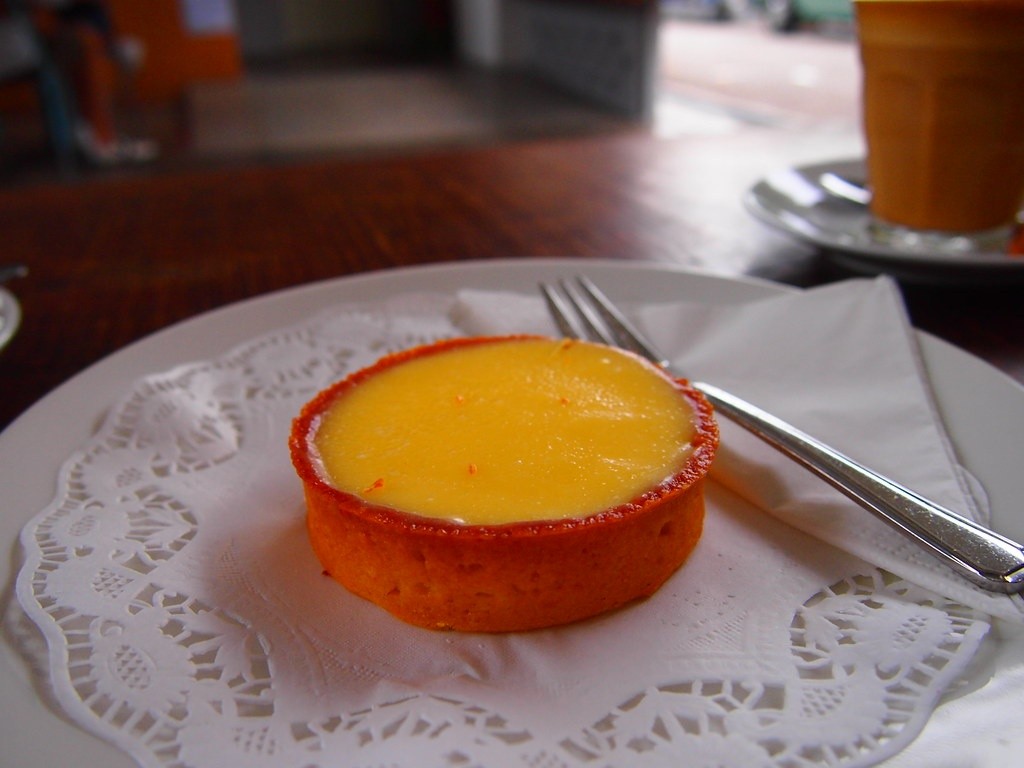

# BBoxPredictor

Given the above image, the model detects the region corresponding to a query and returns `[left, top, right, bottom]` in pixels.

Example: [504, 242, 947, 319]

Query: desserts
[287, 332, 719, 632]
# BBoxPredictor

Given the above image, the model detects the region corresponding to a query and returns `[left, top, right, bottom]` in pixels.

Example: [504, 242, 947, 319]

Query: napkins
[451, 270, 1024, 624]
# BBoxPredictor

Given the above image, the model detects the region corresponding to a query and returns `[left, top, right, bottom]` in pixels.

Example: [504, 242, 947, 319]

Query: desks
[0, 18, 1024, 436]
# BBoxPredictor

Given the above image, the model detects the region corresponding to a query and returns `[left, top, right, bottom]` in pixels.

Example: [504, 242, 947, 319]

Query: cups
[854, 0, 1024, 233]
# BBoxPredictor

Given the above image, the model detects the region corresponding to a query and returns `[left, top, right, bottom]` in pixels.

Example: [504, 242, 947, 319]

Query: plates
[747, 157, 1015, 261]
[0, 259, 1024, 768]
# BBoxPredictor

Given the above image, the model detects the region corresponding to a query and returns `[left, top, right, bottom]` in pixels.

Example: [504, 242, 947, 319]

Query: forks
[540, 274, 1024, 593]
